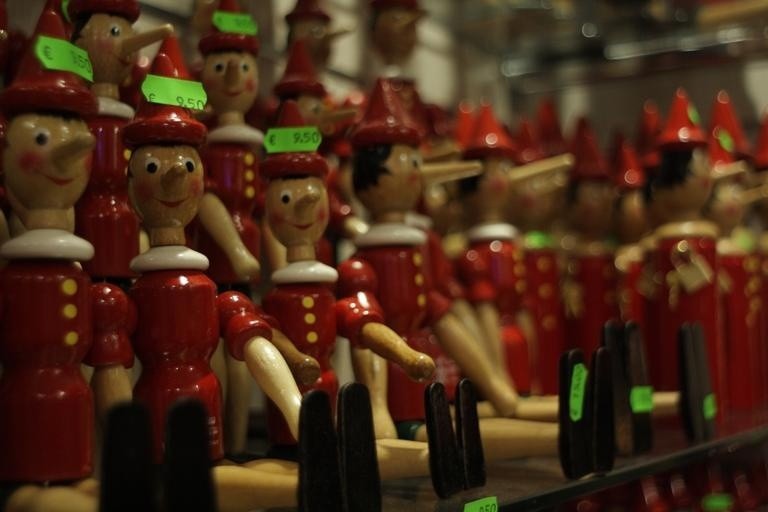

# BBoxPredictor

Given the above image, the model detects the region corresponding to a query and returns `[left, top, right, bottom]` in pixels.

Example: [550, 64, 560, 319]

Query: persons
[0, 0, 766, 512]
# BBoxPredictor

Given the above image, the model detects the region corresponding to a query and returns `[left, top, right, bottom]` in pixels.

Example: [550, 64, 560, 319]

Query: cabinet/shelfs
[1, 1, 768, 512]
